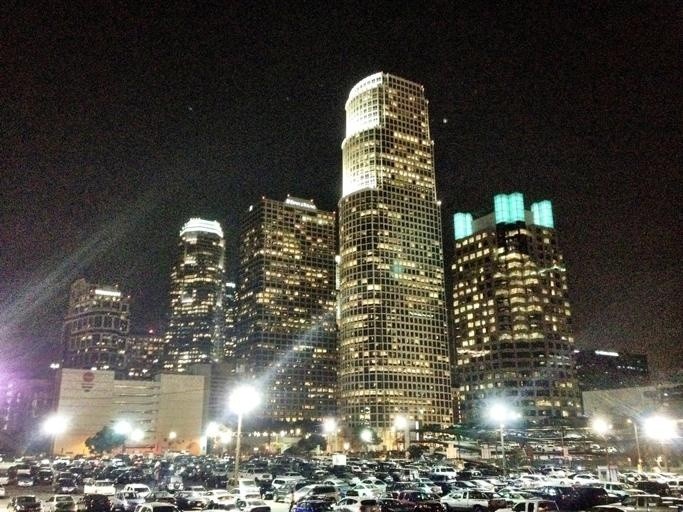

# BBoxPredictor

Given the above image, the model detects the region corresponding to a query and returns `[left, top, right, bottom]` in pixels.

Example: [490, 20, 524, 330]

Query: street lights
[228, 387, 260, 486]
[626, 418, 642, 471]
[488, 405, 511, 476]
[594, 419, 612, 482]
